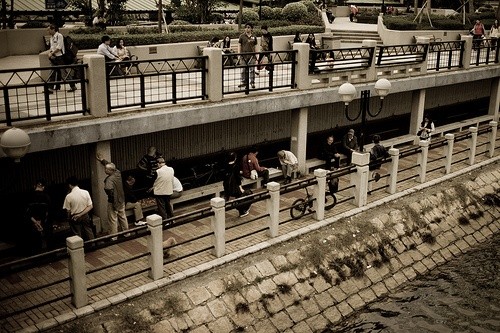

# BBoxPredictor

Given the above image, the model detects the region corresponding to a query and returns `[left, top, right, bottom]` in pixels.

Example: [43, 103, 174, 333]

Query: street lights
[338, 78, 391, 153]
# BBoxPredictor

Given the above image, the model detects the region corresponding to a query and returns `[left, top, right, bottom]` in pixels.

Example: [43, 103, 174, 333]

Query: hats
[261, 25, 268, 31]
[245, 23, 254, 29]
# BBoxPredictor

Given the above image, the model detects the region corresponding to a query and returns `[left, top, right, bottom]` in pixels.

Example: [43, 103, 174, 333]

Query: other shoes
[238, 83, 246, 87]
[239, 204, 253, 218]
[53, 86, 60, 90]
[367, 192, 372, 195]
[67, 88, 77, 92]
[375, 172, 380, 183]
[250, 82, 255, 88]
[255, 72, 260, 76]
[283, 179, 292, 185]
[42, 89, 53, 94]
[134, 221, 147, 226]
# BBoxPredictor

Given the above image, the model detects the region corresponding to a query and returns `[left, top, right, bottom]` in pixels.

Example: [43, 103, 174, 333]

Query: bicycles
[290, 177, 340, 221]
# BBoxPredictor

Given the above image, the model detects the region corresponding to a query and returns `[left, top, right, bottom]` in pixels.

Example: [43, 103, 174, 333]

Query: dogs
[163, 235, 179, 258]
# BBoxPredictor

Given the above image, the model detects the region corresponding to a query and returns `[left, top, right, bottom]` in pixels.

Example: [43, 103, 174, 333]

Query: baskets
[328, 177, 339, 194]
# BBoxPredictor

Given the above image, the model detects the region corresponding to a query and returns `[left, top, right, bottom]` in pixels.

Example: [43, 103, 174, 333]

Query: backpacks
[57, 33, 78, 58]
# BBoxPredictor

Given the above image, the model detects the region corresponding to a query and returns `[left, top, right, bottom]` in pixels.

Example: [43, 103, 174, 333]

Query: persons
[95, 153, 130, 240]
[208, 37, 219, 48]
[317, 129, 358, 170]
[294, 30, 322, 60]
[348, 5, 356, 22]
[113, 38, 132, 75]
[237, 23, 257, 88]
[62, 176, 95, 248]
[416, 114, 435, 141]
[94, 14, 106, 30]
[43, 24, 77, 94]
[223, 151, 250, 217]
[255, 23, 274, 70]
[278, 150, 298, 184]
[98, 36, 119, 77]
[123, 147, 183, 227]
[326, 9, 335, 23]
[23, 181, 49, 248]
[469, 19, 500, 50]
[367, 135, 389, 195]
[386, 6, 394, 15]
[239, 146, 270, 188]
[221, 35, 240, 66]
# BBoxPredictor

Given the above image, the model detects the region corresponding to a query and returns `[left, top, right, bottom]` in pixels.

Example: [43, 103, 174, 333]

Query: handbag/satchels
[250, 169, 259, 180]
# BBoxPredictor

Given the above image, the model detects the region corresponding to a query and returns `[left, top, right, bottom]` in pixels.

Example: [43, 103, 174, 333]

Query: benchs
[413, 34, 443, 53]
[306, 134, 415, 169]
[124, 167, 282, 217]
[72, 49, 139, 77]
[431, 115, 494, 138]
[0, 214, 101, 251]
[287, 40, 322, 61]
[196, 44, 241, 68]
[459, 33, 487, 49]
